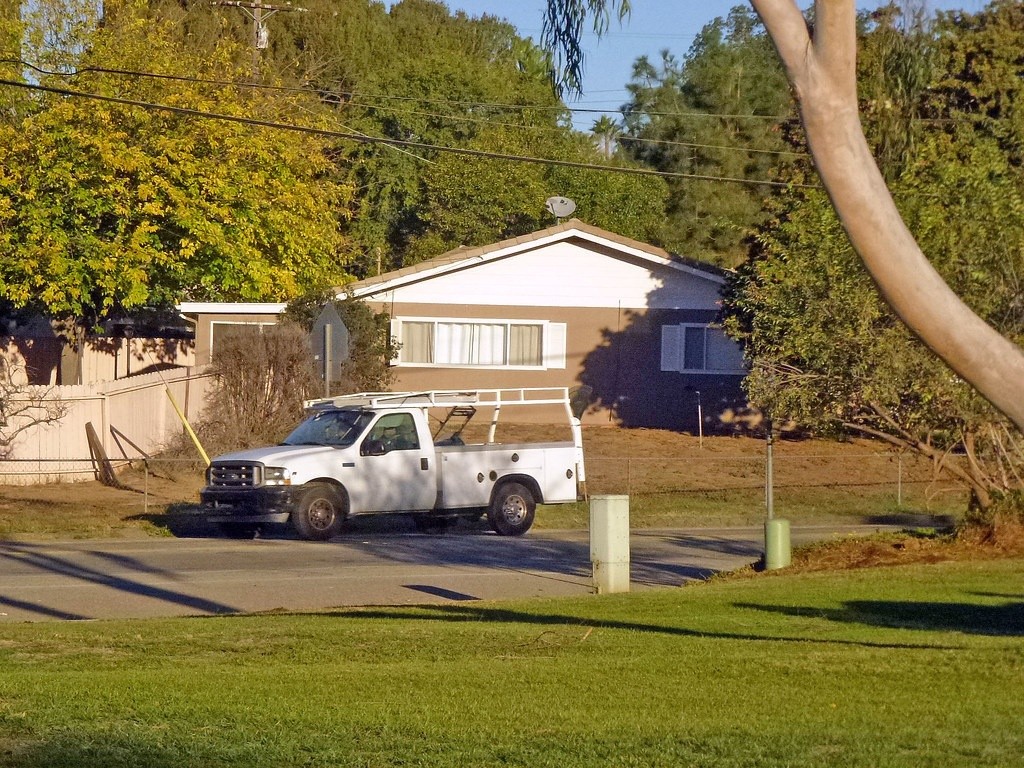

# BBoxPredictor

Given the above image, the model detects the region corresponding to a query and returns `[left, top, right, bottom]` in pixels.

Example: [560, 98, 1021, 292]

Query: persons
[337, 423, 356, 444]
[373, 426, 414, 452]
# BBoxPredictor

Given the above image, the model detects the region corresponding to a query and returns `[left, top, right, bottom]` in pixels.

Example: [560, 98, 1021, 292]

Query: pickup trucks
[200, 387, 586, 541]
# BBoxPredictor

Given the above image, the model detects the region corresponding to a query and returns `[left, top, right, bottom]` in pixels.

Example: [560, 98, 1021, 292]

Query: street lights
[122, 323, 135, 377]
